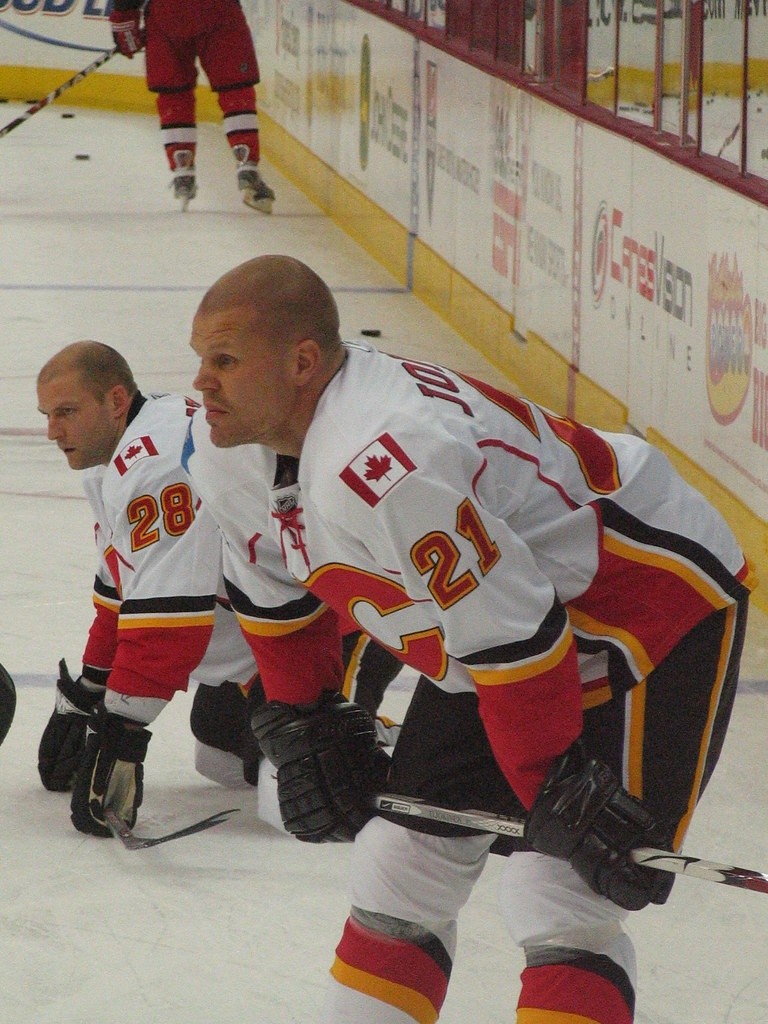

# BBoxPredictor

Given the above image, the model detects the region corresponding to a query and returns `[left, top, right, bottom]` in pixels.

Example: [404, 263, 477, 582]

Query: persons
[110, 0, 275, 215]
[37, 340, 404, 839]
[178, 256, 758, 1024]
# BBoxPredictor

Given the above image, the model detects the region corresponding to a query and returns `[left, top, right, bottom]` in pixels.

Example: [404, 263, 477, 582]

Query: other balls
[27, 99, 38, 104]
[76, 154, 89, 160]
[359, 328, 380, 338]
[61, 112, 74, 119]
[0, 98, 8, 102]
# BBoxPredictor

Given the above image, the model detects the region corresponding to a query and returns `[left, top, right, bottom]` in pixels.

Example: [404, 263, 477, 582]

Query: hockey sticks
[0, 31, 145, 138]
[101, 807, 244, 851]
[367, 789, 768, 897]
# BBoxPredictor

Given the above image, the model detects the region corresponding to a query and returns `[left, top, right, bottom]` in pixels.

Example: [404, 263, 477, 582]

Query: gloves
[249, 692, 396, 850]
[534, 742, 682, 913]
[36, 659, 114, 797]
[74, 702, 153, 846]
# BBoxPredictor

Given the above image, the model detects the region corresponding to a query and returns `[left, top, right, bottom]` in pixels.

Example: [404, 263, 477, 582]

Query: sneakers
[171, 167, 199, 215]
[235, 162, 277, 215]
